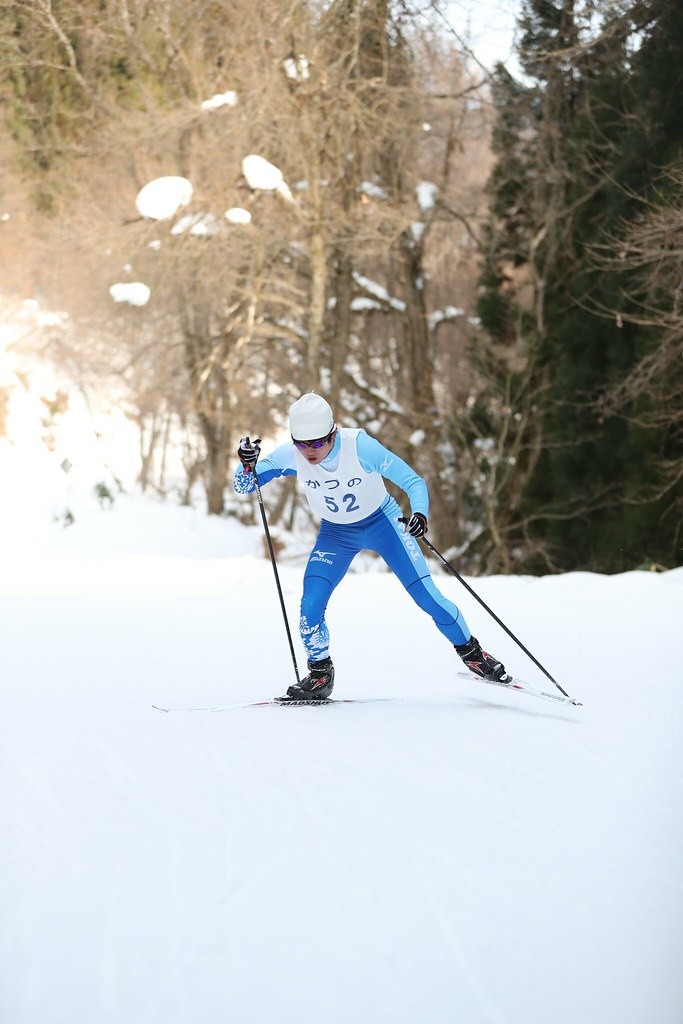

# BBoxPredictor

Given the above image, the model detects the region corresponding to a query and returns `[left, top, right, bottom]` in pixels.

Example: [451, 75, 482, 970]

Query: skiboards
[151, 670, 578, 713]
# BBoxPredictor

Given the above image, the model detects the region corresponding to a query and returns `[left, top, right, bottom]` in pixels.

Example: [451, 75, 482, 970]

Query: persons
[234, 393, 513, 702]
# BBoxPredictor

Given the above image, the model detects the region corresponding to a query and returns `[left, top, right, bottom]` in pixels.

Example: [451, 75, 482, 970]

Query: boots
[287, 655, 335, 699]
[454, 635, 504, 678]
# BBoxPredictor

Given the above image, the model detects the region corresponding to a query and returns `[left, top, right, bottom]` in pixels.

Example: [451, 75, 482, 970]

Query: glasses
[294, 438, 326, 451]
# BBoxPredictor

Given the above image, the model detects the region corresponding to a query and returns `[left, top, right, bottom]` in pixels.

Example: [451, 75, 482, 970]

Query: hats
[289, 392, 334, 441]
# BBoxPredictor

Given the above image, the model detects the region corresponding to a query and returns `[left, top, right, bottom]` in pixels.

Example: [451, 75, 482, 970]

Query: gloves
[403, 514, 427, 538]
[238, 438, 261, 472]
